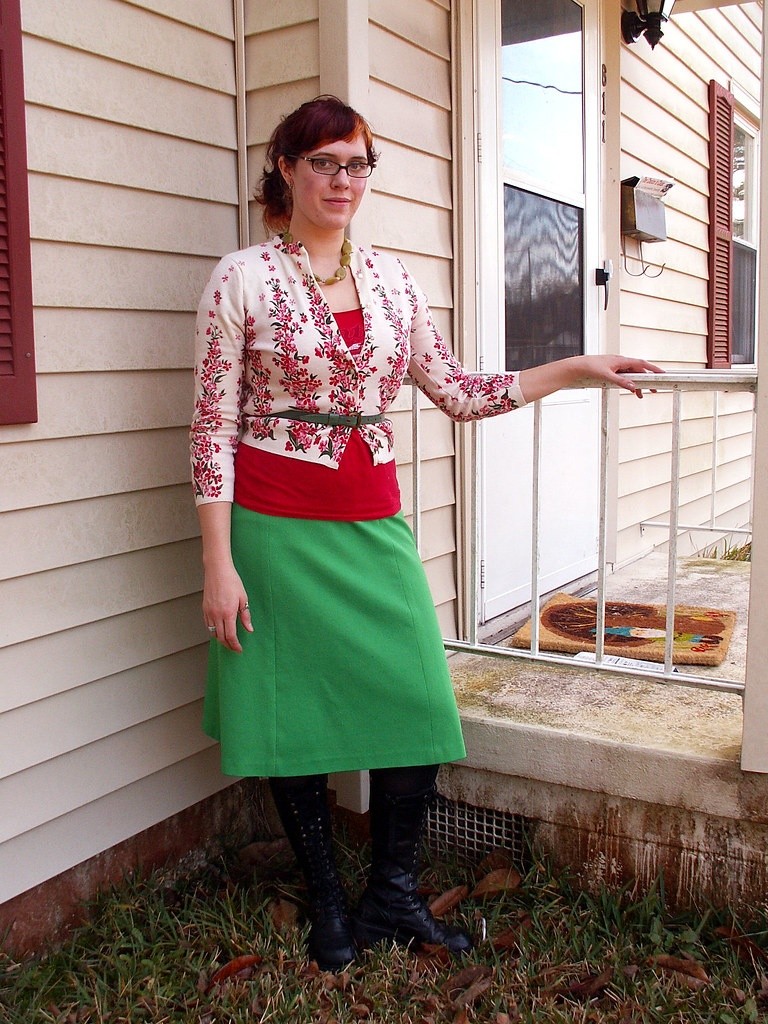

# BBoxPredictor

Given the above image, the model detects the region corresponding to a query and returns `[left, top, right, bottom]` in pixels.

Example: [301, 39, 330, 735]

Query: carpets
[510, 593, 737, 667]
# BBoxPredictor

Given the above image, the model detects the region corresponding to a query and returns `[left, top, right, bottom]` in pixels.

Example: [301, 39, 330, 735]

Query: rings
[207, 625, 217, 631]
[236, 601, 250, 614]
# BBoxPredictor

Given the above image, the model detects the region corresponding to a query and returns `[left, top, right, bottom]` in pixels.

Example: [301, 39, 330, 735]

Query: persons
[190, 96, 670, 971]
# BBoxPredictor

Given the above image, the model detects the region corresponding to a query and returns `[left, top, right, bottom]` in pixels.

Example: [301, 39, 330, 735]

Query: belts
[269, 411, 385, 429]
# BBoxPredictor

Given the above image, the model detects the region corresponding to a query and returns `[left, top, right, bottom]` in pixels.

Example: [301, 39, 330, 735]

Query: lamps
[621, 0, 676, 49]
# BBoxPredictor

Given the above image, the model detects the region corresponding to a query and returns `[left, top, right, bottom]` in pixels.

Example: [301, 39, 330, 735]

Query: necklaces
[282, 232, 354, 284]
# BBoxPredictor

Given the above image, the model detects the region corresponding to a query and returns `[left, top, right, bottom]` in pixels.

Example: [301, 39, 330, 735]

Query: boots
[271, 781, 353, 968]
[353, 781, 473, 957]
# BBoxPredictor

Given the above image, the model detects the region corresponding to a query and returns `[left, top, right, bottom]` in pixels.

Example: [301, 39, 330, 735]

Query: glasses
[288, 154, 377, 178]
